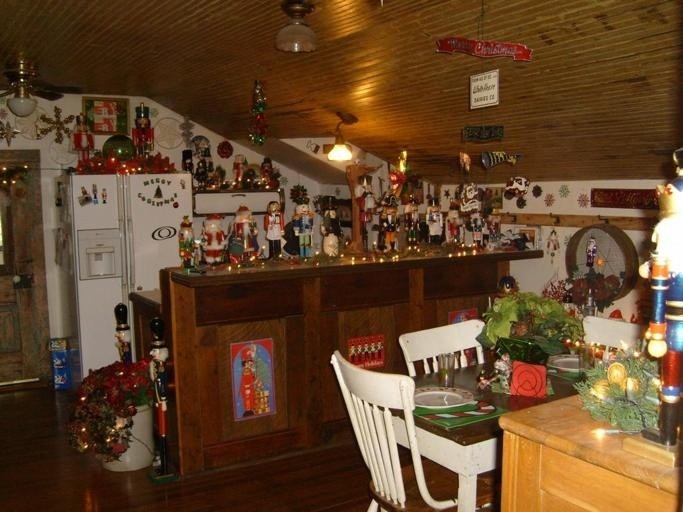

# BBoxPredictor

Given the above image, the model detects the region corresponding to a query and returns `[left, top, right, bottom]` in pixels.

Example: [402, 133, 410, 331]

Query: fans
[1, 57, 68, 103]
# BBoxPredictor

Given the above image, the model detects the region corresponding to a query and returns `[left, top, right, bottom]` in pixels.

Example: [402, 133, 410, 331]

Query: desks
[496, 383, 682, 512]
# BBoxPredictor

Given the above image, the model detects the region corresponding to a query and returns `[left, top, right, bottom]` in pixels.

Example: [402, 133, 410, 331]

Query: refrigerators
[55, 173, 194, 395]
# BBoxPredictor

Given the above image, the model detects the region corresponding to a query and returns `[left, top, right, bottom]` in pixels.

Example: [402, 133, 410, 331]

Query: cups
[438, 354, 453, 388]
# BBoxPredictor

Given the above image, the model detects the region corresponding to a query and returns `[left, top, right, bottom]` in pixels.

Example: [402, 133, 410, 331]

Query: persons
[70, 112, 95, 175]
[639, 146, 683, 445]
[146, 315, 173, 473]
[111, 303, 135, 364]
[132, 101, 154, 160]
[177, 154, 491, 268]
[585, 236, 598, 270]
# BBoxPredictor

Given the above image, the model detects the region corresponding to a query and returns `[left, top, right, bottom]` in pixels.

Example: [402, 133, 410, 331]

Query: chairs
[580, 314, 651, 353]
[394, 318, 489, 379]
[328, 349, 495, 512]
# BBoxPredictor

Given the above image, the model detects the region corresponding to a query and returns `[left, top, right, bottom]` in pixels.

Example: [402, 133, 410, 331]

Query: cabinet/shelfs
[192, 189, 285, 259]
[127, 243, 546, 496]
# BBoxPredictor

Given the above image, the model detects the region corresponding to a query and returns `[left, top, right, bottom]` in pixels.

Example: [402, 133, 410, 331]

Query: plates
[545, 352, 589, 372]
[411, 386, 471, 409]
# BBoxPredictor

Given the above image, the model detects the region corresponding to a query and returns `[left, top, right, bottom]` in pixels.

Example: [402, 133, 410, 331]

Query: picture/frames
[79, 93, 131, 138]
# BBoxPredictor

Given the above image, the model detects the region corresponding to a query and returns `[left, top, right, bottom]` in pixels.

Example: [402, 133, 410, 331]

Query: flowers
[543, 267, 625, 314]
[64, 354, 151, 464]
[475, 290, 590, 361]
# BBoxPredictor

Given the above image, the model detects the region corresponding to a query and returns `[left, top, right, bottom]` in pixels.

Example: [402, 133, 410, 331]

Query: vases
[494, 336, 549, 367]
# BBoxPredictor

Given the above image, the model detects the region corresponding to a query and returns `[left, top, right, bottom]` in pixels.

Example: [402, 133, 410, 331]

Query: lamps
[5, 94, 39, 117]
[270, 0, 321, 55]
[326, 110, 358, 160]
